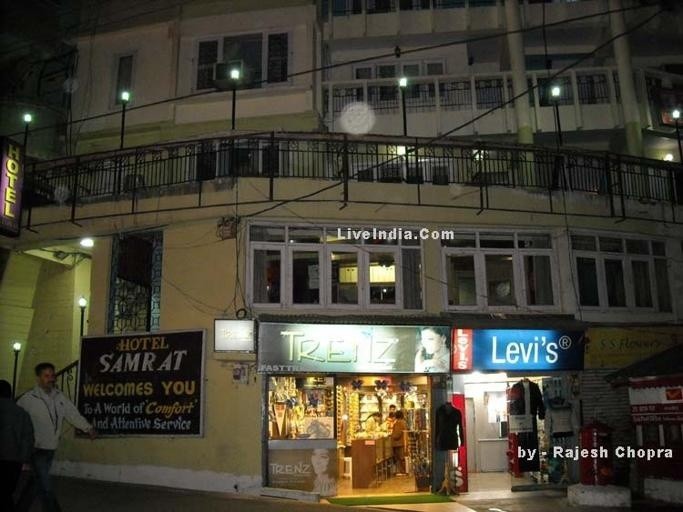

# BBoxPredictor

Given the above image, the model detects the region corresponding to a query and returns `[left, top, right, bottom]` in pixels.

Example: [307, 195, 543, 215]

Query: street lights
[22, 113, 32, 155]
[228, 68, 241, 130]
[120, 90, 129, 149]
[551, 86, 563, 146]
[397, 76, 407, 137]
[11, 342, 22, 401]
[78, 297, 87, 337]
[671, 109, 682, 162]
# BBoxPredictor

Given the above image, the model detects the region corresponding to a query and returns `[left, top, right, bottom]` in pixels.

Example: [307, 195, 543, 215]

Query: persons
[311, 448, 337, 496]
[414, 327, 450, 373]
[435, 401, 464, 451]
[365, 404, 407, 476]
[0, 363, 98, 512]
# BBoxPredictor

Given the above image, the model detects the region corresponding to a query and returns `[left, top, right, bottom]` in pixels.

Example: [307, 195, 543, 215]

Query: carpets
[326, 494, 456, 506]
